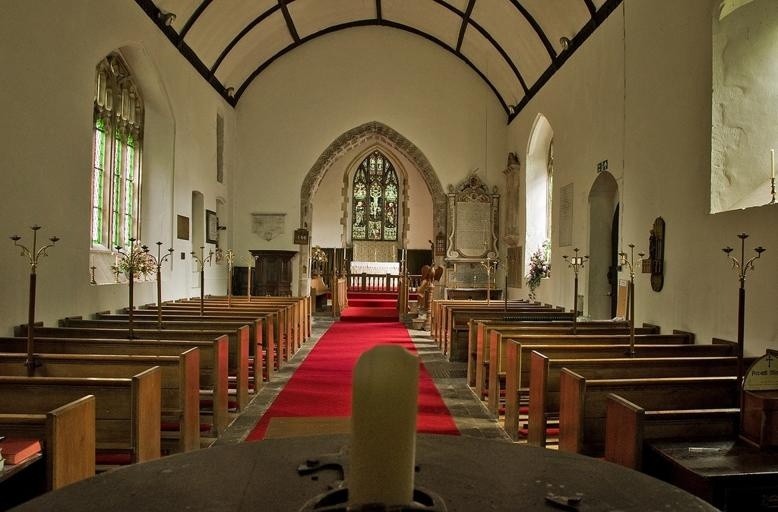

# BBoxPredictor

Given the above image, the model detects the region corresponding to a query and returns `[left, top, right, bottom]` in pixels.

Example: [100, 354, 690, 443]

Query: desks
[2, 435, 716, 507]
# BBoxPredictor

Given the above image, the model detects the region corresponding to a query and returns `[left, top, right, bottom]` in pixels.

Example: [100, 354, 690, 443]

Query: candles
[92, 255, 95, 266]
[116, 256, 119, 267]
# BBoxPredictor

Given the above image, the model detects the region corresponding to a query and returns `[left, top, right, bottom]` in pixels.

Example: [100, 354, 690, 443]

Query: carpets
[243, 286, 462, 441]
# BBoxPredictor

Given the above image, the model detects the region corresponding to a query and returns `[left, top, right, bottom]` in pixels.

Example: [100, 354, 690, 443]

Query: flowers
[313, 247, 328, 264]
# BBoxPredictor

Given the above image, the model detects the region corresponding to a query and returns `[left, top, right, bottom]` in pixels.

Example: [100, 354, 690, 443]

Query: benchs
[2, 296, 313, 492]
[432, 297, 757, 470]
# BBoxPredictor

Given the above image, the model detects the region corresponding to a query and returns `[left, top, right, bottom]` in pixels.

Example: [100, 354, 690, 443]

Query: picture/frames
[206, 210, 217, 244]
